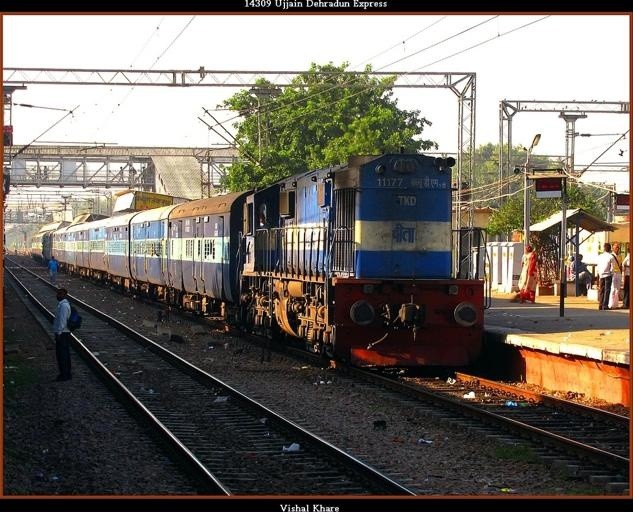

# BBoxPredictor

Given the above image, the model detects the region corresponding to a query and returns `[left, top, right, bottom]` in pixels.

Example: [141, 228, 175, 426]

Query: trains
[32, 146, 485, 370]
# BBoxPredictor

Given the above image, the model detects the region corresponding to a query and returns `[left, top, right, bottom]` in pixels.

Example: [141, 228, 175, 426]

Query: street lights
[524, 133, 542, 247]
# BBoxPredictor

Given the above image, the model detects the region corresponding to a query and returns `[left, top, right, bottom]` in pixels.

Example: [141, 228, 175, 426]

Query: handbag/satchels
[67, 307, 81, 329]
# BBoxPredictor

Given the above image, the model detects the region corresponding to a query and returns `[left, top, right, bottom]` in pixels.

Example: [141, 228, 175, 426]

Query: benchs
[557, 282, 584, 296]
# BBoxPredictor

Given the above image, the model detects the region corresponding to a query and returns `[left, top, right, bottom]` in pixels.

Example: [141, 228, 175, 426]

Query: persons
[570, 254, 593, 290]
[597, 243, 614, 310]
[621, 245, 630, 309]
[48, 256, 59, 285]
[518, 246, 538, 304]
[608, 243, 622, 309]
[51, 288, 72, 382]
